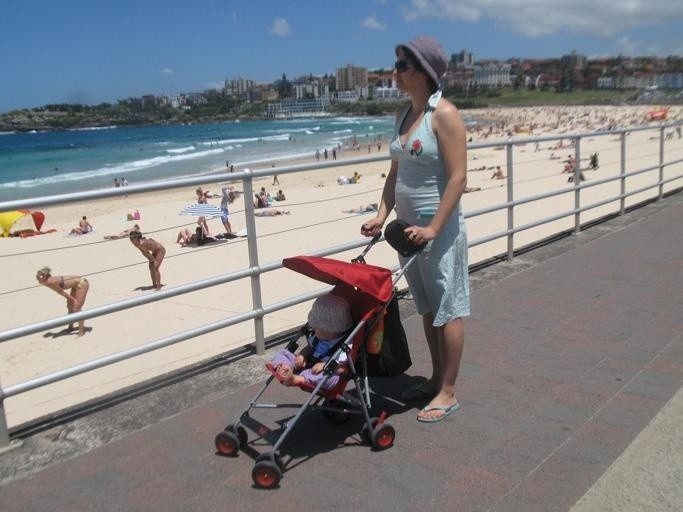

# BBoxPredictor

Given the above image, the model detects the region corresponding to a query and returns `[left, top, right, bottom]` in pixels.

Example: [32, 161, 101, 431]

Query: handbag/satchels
[383, 219, 427, 258]
[355, 291, 413, 379]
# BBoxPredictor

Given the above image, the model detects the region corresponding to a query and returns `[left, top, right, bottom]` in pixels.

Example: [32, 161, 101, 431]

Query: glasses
[393, 61, 420, 73]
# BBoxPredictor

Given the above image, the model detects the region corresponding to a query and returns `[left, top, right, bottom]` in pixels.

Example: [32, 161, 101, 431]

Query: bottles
[364, 319, 384, 355]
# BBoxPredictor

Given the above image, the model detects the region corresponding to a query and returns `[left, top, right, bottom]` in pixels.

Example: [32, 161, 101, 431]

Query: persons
[113, 178, 129, 187]
[129, 231, 166, 291]
[35, 266, 90, 338]
[338, 171, 363, 185]
[464, 131, 601, 192]
[315, 134, 382, 162]
[70, 215, 143, 241]
[177, 159, 286, 244]
[272, 293, 356, 387]
[358, 34, 471, 423]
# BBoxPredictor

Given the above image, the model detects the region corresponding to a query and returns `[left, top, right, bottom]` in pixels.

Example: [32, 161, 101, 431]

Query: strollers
[213, 225, 428, 489]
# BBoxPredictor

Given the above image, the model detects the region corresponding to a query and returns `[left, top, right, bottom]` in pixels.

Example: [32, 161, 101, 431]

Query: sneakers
[264, 359, 293, 387]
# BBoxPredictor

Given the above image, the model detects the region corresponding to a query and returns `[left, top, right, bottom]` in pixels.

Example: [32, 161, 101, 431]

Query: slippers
[414, 393, 461, 425]
[403, 381, 437, 402]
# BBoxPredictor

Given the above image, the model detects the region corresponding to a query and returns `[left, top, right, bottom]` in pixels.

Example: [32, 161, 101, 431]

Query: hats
[393, 33, 449, 92]
[305, 293, 354, 334]
